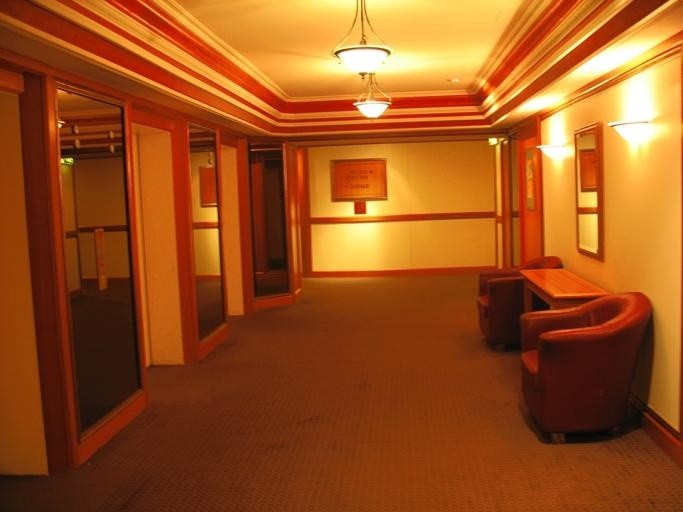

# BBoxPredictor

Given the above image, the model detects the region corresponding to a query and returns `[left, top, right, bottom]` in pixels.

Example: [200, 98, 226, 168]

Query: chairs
[475, 255, 652, 444]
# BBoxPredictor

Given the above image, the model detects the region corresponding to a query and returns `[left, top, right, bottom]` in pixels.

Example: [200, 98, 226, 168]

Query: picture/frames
[329, 159, 387, 201]
[579, 148, 598, 192]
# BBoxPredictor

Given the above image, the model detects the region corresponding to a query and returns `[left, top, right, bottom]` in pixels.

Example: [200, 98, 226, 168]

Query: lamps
[536, 144, 563, 161]
[332, 1, 392, 122]
[608, 119, 650, 142]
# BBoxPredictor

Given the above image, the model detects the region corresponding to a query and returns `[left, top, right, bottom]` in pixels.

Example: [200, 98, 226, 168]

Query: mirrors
[54, 79, 146, 442]
[573, 121, 605, 263]
[187, 122, 226, 342]
